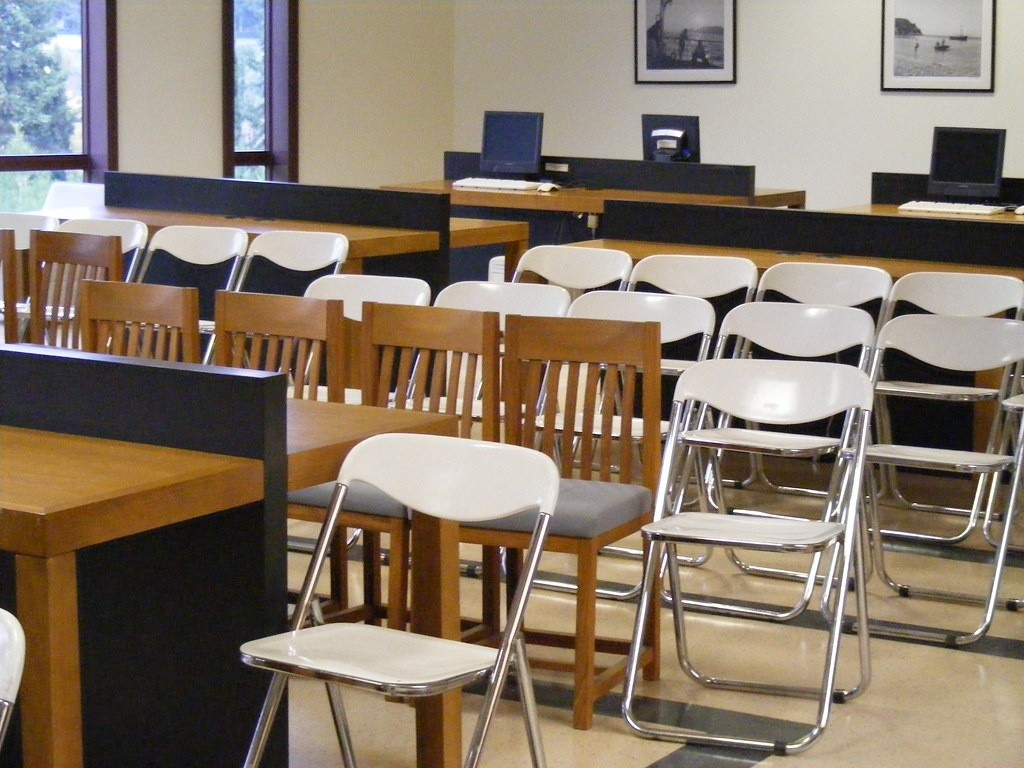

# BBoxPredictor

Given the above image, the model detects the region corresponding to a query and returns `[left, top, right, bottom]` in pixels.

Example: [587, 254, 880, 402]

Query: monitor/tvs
[642, 114, 701, 163]
[480, 110, 544, 174]
[929, 127, 1007, 198]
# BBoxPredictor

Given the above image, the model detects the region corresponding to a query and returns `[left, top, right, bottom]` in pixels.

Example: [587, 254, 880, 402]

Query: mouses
[1014, 205, 1024, 215]
[538, 183, 559, 192]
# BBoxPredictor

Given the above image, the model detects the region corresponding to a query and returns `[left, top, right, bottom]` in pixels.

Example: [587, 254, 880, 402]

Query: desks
[839, 171, 1024, 227]
[0, 342, 465, 768]
[558, 198, 1024, 520]
[60, 168, 530, 284]
[380, 149, 806, 290]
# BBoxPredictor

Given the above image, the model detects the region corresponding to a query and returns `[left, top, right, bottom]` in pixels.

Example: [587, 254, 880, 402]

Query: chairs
[406, 314, 660, 731]
[0, 211, 431, 410]
[431, 243, 1022, 624]
[283, 300, 500, 705]
[214, 288, 350, 616]
[1, 608, 26, 748]
[819, 313, 1024, 650]
[238, 432, 561, 768]
[621, 359, 874, 756]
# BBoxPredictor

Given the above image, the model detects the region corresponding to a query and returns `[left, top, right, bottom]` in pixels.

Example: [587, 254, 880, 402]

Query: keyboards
[897, 200, 1006, 215]
[452, 177, 545, 191]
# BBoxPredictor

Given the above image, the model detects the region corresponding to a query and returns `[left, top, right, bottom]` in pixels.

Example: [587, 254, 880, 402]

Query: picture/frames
[634, 0, 737, 85]
[881, 0, 997, 93]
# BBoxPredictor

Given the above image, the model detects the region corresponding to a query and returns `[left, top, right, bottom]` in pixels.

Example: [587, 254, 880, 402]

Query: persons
[647, 19, 661, 58]
[677, 28, 691, 57]
[691, 38, 706, 63]
